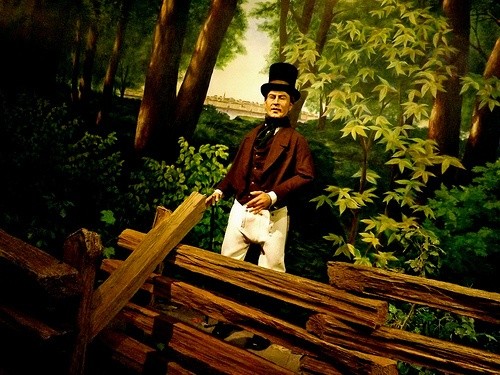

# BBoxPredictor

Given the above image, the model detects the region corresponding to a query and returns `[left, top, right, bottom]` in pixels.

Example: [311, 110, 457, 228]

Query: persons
[204, 60, 318, 353]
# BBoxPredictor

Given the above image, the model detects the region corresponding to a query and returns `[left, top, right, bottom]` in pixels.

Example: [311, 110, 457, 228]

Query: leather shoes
[212, 319, 235, 339]
[249, 327, 273, 351]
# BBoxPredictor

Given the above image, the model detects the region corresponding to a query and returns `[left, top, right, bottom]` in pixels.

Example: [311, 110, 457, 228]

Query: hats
[261, 62, 301, 102]
